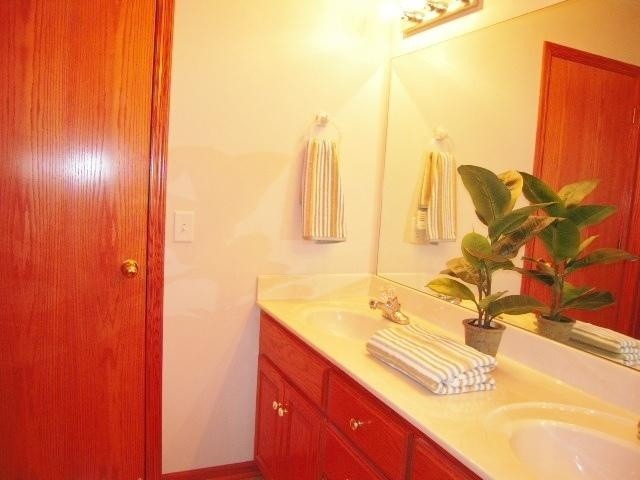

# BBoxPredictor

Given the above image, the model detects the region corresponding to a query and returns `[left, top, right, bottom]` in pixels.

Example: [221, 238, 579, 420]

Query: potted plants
[504, 170, 640, 345]
[423, 162, 566, 357]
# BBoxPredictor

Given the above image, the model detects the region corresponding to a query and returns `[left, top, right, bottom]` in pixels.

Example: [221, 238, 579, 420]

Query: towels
[365, 322, 498, 396]
[407, 149, 459, 245]
[298, 135, 348, 242]
[568, 321, 640, 368]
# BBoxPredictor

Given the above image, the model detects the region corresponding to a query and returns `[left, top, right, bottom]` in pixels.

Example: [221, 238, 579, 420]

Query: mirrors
[374, 0, 640, 373]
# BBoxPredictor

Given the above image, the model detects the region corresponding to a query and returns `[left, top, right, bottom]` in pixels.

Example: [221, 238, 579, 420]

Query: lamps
[377, 0, 484, 40]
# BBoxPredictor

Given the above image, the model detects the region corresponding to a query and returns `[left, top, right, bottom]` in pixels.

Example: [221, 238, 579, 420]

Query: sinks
[313, 309, 384, 344]
[507, 417, 640, 480]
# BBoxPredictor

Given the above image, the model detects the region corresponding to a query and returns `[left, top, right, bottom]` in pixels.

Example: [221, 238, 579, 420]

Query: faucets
[369, 295, 410, 325]
[439, 294, 461, 305]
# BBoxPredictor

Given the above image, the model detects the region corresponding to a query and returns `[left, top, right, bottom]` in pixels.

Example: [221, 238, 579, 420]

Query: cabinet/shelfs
[253, 308, 486, 480]
